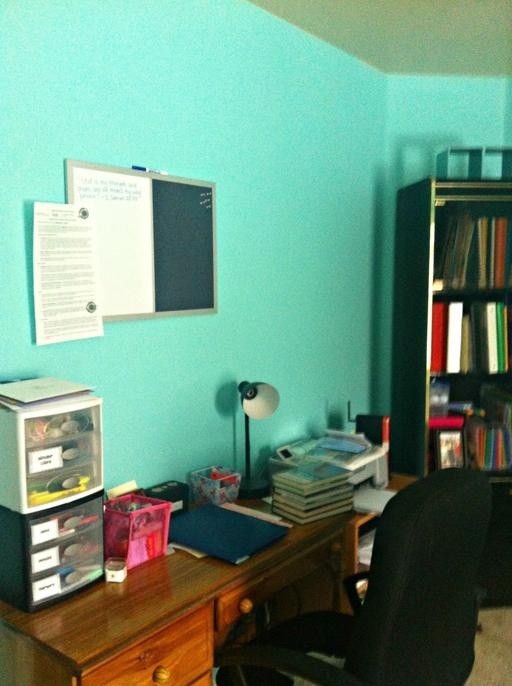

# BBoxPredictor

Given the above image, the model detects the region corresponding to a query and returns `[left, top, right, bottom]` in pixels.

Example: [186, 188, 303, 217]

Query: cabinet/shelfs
[1, 375, 106, 614]
[347, 474, 413, 606]
[1, 548, 215, 684]
[215, 495, 349, 642]
[391, 175, 512, 609]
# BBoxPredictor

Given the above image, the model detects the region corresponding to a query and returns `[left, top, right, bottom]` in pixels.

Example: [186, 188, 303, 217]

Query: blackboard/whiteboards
[65, 159, 217, 323]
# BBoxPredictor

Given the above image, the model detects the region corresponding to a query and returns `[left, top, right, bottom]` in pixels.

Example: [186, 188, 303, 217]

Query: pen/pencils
[132, 166, 169, 176]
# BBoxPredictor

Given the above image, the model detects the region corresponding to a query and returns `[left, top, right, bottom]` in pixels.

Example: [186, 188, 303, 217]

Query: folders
[169, 502, 289, 565]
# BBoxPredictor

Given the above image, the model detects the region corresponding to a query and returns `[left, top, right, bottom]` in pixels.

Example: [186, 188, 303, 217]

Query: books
[270, 460, 356, 526]
[428, 213, 511, 472]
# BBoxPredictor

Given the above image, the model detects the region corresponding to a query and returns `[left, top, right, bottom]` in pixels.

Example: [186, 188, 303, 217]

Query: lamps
[234, 382, 281, 499]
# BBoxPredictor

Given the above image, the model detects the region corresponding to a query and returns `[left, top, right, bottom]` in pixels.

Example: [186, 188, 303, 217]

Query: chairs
[245, 583, 477, 684]
[214, 468, 493, 685]
